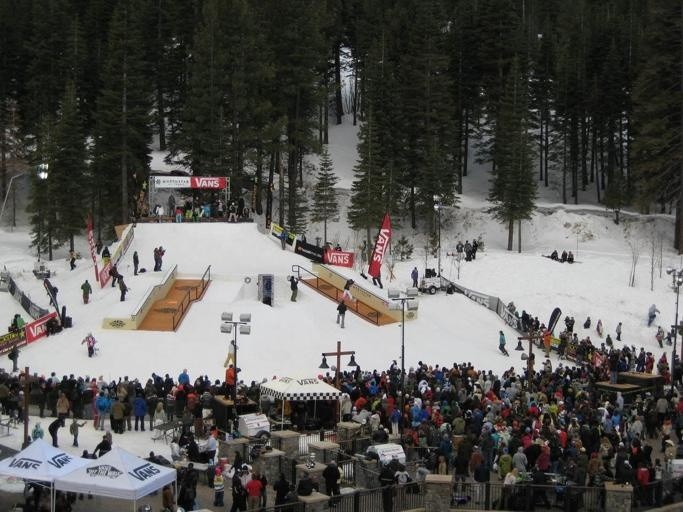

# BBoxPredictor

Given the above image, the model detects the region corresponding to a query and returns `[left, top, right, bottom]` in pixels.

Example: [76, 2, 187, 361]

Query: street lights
[385, 287, 420, 421]
[432, 200, 445, 277]
[217, 311, 254, 402]
[318, 339, 358, 391]
[666, 266, 682, 394]
[0, 162, 50, 221]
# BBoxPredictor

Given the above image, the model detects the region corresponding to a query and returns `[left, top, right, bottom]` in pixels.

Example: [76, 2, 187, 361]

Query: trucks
[594, 369, 663, 409]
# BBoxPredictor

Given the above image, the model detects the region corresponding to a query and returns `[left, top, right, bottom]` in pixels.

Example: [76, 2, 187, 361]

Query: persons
[342, 279, 354, 301]
[280, 229, 287, 250]
[410, 266, 417, 288]
[336, 299, 346, 328]
[68, 250, 75, 271]
[372, 273, 383, 289]
[289, 276, 297, 301]
[550, 250, 558, 260]
[95, 239, 165, 301]
[80, 279, 92, 304]
[0, 308, 681, 510]
[456, 238, 478, 261]
[646, 304, 660, 327]
[561, 250, 567, 262]
[152, 192, 244, 222]
[46, 287, 58, 307]
[334, 243, 341, 252]
[567, 251, 573, 264]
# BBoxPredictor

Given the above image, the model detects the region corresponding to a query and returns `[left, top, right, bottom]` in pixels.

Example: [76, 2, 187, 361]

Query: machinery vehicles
[419, 277, 444, 295]
[33, 261, 50, 279]
